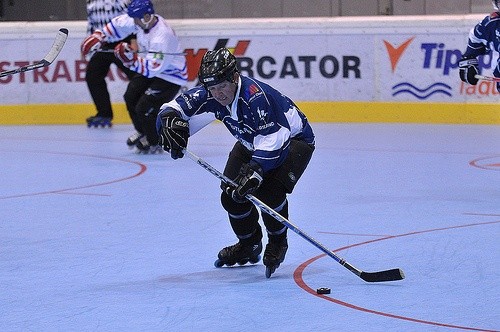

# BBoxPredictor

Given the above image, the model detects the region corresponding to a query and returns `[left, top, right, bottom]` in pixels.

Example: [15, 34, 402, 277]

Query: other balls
[317, 287, 331, 294]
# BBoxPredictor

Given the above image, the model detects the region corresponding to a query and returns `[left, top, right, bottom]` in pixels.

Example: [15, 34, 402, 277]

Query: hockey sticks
[0, 28, 69, 76]
[474, 74, 499, 82]
[92, 49, 188, 56]
[179, 146, 404, 282]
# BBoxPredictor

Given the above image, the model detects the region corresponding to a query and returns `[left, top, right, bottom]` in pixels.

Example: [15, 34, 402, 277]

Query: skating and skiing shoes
[126, 131, 144, 148]
[213, 234, 263, 268]
[262, 237, 288, 278]
[86, 112, 113, 128]
[134, 136, 160, 154]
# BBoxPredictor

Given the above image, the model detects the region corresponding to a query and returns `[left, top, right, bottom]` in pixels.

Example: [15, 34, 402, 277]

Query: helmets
[491, 0, 500, 12]
[127, 0, 154, 19]
[198, 48, 239, 88]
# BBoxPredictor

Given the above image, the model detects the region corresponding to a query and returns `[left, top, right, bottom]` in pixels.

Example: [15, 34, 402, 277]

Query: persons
[155, 48, 316, 265]
[80, 0, 188, 150]
[459, 0, 500, 97]
[86, 0, 136, 125]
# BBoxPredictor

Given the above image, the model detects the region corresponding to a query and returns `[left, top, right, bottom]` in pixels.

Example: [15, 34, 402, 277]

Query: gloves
[113, 42, 138, 65]
[221, 164, 264, 203]
[81, 29, 106, 62]
[157, 112, 190, 160]
[457, 58, 480, 85]
